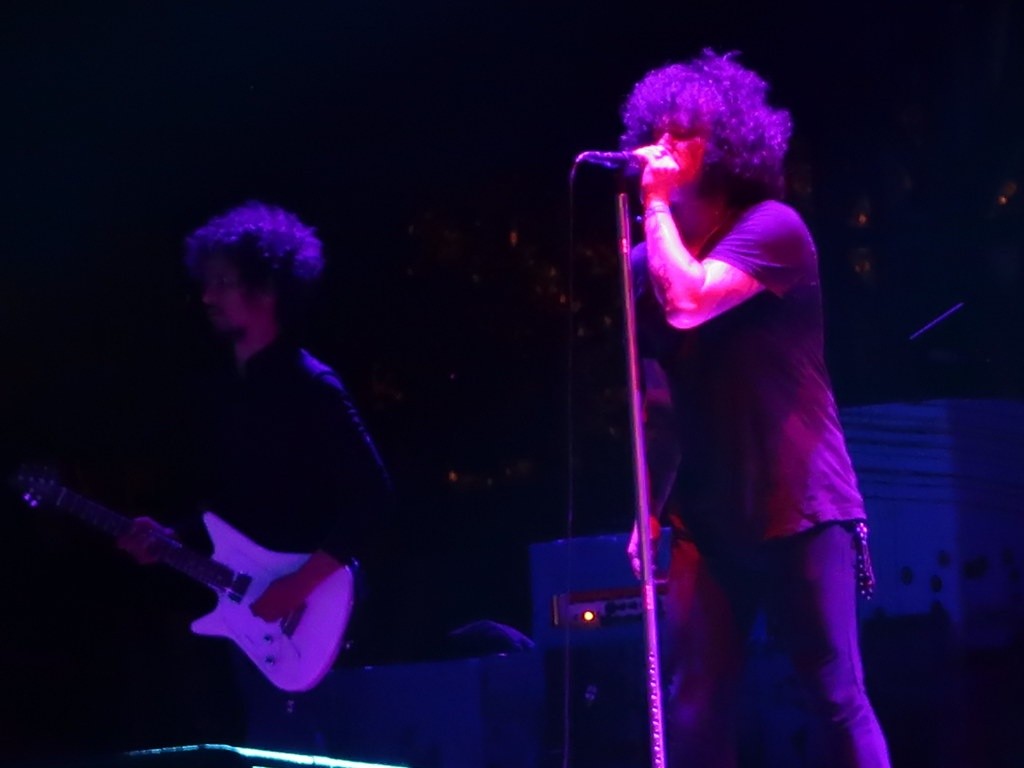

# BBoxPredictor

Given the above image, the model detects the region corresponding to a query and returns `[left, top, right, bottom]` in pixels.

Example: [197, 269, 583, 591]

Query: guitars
[17, 457, 369, 695]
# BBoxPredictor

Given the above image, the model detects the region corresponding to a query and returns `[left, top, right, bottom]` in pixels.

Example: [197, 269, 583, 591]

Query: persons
[615, 51, 892, 768]
[120, 211, 389, 749]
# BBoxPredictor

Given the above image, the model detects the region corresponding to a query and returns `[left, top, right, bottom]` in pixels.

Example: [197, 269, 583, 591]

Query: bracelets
[637, 203, 669, 221]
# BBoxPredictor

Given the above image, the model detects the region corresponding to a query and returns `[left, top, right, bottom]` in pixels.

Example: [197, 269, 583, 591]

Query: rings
[654, 146, 666, 159]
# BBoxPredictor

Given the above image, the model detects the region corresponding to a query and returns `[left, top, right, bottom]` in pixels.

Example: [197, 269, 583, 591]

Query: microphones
[587, 140, 654, 187]
